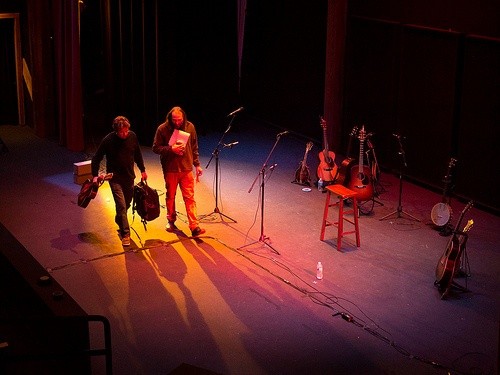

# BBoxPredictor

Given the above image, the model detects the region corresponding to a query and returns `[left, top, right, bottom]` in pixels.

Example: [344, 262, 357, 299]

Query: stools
[320, 184, 359, 250]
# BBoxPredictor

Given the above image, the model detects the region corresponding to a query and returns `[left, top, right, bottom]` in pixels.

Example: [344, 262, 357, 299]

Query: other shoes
[191, 227, 206, 237]
[122, 235, 131, 245]
[166, 222, 174, 230]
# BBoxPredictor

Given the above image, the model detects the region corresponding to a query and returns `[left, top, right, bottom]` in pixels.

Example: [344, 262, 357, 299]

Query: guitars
[431, 157, 458, 226]
[367, 141, 384, 193]
[77, 172, 114, 209]
[435, 199, 474, 283]
[346, 130, 375, 201]
[295, 142, 314, 183]
[315, 119, 339, 182]
[336, 126, 358, 186]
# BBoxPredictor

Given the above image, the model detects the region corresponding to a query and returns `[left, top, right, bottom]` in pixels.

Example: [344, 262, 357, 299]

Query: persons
[153, 107, 205, 238]
[91, 116, 147, 246]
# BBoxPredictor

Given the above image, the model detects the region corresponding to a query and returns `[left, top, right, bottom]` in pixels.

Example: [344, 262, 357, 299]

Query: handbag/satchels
[132, 178, 160, 233]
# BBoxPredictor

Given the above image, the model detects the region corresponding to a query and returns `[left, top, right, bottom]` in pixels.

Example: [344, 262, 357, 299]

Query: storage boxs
[73, 160, 93, 184]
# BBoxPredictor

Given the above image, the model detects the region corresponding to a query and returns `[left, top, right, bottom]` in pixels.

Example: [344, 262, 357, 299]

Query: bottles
[317, 261, 323, 280]
[318, 178, 324, 191]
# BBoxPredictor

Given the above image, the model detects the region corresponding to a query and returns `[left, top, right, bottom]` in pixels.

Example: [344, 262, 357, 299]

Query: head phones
[167, 108, 186, 122]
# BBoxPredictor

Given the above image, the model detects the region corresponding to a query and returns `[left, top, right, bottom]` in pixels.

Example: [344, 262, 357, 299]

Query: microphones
[277, 130, 289, 137]
[228, 106, 244, 116]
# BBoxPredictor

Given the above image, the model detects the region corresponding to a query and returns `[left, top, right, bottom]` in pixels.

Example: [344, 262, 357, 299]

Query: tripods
[236, 134, 282, 255]
[379, 135, 421, 222]
[197, 113, 237, 223]
